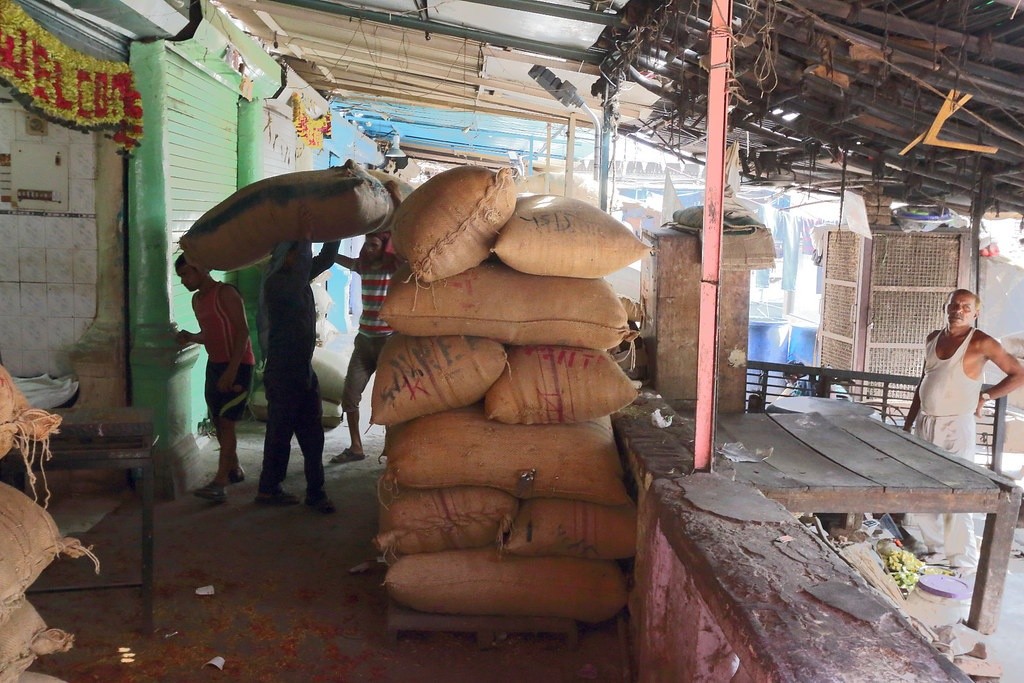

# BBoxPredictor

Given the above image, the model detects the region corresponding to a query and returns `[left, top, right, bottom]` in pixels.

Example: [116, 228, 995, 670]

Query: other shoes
[228, 465, 245, 483]
[193, 482, 226, 502]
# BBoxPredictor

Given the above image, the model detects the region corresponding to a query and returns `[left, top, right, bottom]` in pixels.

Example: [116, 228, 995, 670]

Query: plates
[889, 565, 960, 589]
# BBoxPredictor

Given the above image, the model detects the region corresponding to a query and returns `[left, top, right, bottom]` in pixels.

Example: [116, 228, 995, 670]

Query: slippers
[254, 486, 299, 504]
[330, 448, 366, 463]
[305, 492, 335, 513]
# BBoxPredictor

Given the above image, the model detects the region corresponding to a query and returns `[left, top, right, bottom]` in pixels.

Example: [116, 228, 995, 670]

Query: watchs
[980, 390, 991, 402]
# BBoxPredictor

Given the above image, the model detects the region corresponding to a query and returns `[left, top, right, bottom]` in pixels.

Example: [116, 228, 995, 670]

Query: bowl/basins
[890, 205, 957, 232]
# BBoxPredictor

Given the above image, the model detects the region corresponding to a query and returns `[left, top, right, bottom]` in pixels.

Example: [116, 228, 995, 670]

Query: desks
[0, 405, 154, 637]
[682, 411, 1022, 633]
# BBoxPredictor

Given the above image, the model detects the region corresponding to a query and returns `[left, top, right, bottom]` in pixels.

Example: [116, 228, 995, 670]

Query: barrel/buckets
[905, 574, 973, 628]
[747, 317, 790, 395]
[788, 325, 818, 366]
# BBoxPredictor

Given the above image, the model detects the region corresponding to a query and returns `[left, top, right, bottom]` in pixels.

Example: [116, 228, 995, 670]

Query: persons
[903, 289, 1024, 570]
[258, 238, 341, 515]
[332, 230, 403, 463]
[174, 252, 255, 504]
[774, 360, 855, 402]
